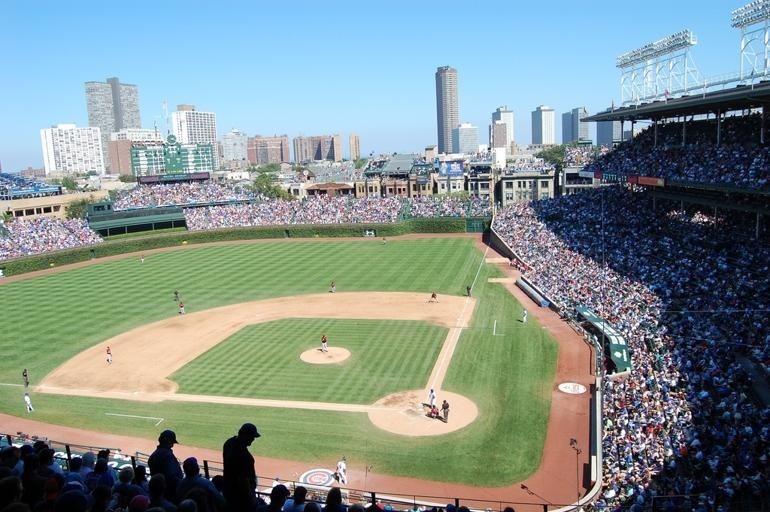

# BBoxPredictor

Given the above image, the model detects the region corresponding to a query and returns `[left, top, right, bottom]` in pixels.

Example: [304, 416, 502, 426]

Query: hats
[82, 452, 97, 464]
[238, 423, 261, 439]
[158, 430, 179, 444]
[128, 494, 151, 511]
[269, 485, 291, 499]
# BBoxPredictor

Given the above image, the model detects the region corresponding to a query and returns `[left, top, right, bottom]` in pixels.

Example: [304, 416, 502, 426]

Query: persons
[0, 114, 769, 511]
